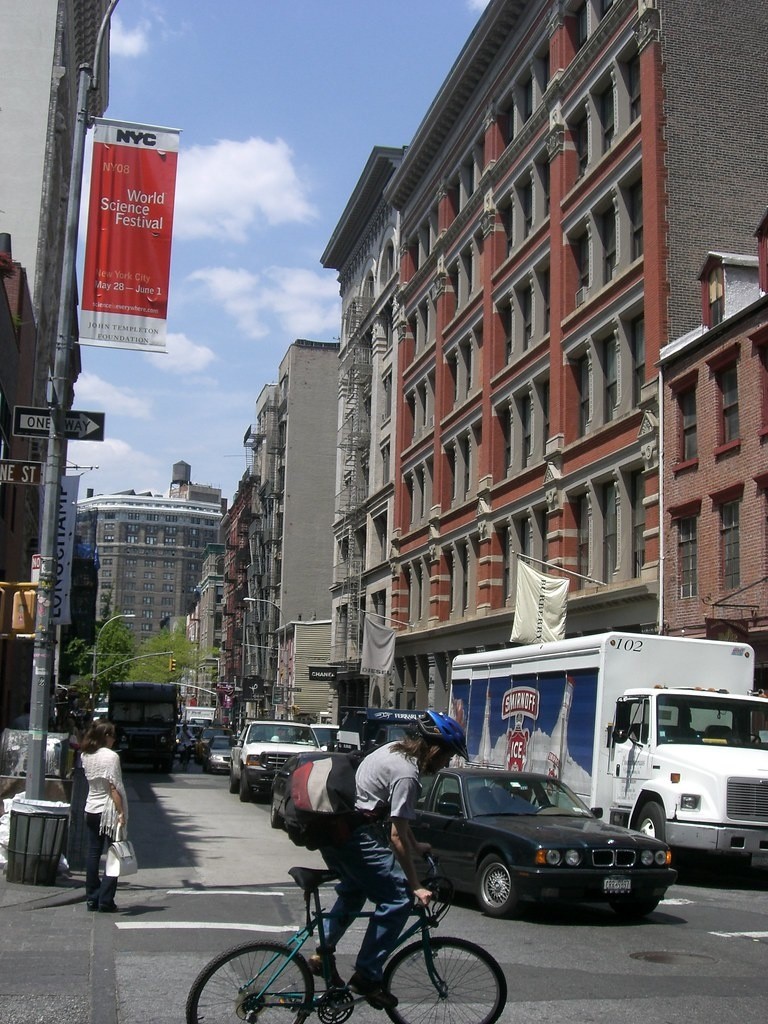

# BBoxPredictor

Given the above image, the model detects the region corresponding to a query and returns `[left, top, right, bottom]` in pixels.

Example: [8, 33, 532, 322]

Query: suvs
[192, 725, 234, 766]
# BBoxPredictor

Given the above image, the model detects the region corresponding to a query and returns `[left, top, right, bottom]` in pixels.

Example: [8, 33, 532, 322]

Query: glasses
[107, 732, 116, 739]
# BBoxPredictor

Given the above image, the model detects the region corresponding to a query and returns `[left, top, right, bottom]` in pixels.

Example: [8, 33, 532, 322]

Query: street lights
[244, 598, 287, 721]
[93, 614, 137, 710]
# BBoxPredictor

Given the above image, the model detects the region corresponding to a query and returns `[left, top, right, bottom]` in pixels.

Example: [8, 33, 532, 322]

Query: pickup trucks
[228, 720, 329, 805]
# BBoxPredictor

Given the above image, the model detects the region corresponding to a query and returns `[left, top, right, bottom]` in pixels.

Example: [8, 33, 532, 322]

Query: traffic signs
[12, 406, 106, 442]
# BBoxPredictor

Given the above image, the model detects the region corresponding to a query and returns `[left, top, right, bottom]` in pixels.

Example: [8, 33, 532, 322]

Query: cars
[176, 725, 199, 752]
[272, 753, 348, 838]
[402, 768, 678, 922]
[298, 723, 340, 747]
[202, 735, 235, 773]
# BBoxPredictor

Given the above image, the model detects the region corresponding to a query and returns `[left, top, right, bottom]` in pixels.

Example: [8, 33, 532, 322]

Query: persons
[12, 689, 79, 730]
[176, 724, 193, 764]
[80, 718, 128, 912]
[307, 710, 470, 1008]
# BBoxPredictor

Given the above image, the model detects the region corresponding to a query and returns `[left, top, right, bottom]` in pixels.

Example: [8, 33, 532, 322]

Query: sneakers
[308, 946, 346, 989]
[346, 970, 398, 1008]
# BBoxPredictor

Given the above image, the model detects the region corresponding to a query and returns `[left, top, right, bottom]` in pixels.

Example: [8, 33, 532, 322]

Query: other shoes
[87, 899, 99, 910]
[99, 902, 118, 912]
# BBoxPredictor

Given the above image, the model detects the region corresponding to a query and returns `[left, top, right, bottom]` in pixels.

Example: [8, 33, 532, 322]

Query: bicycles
[186, 843, 508, 1024]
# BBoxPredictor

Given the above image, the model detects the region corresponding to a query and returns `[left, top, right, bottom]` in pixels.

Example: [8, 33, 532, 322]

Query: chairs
[437, 792, 461, 815]
[253, 731, 266, 741]
[704, 724, 734, 742]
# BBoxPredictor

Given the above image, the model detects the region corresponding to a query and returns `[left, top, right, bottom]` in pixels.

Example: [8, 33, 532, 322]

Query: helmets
[417, 710, 469, 761]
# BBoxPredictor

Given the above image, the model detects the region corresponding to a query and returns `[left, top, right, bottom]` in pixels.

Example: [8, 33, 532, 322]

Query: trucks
[182, 705, 216, 727]
[446, 630, 768, 864]
[91, 703, 108, 722]
[108, 681, 181, 774]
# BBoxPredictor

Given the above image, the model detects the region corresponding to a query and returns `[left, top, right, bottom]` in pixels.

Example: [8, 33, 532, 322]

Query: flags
[509, 559, 571, 645]
[360, 616, 396, 676]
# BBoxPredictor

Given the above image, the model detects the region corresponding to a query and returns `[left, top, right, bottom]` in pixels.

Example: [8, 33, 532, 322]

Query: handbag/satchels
[283, 754, 356, 849]
[106, 821, 138, 877]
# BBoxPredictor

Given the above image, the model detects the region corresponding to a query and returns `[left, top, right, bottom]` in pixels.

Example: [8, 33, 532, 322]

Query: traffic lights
[169, 657, 177, 672]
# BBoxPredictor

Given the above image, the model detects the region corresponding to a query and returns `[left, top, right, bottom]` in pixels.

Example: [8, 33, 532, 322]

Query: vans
[335, 707, 428, 755]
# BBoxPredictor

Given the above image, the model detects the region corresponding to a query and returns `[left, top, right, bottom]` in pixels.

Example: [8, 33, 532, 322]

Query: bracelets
[118, 813, 126, 819]
